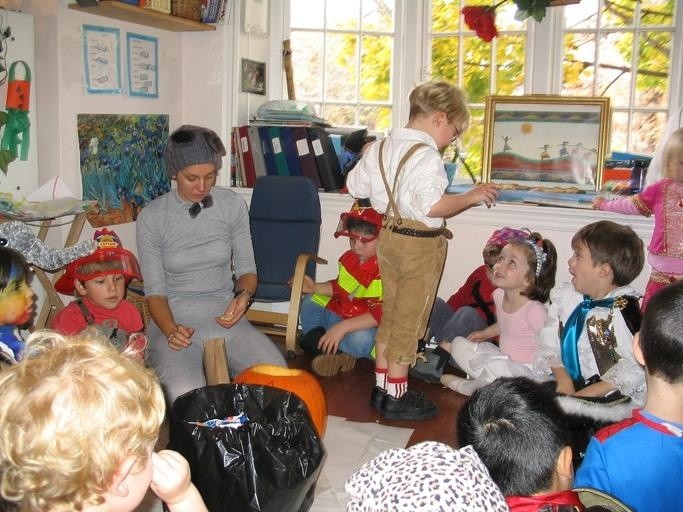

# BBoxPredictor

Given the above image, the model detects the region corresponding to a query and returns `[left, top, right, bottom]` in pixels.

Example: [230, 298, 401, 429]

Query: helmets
[54, 248, 143, 297]
[334, 199, 383, 242]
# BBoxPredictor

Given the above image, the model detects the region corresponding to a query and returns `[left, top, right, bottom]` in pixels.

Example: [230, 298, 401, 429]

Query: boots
[299, 326, 357, 377]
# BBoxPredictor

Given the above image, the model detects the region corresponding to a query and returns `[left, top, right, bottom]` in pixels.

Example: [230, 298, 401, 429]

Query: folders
[240, 122, 345, 192]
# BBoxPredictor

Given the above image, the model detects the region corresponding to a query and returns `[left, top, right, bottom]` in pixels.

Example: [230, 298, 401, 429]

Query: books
[232, 111, 340, 194]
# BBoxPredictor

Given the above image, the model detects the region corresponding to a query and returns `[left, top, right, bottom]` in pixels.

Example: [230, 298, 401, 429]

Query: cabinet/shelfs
[0, 196, 101, 336]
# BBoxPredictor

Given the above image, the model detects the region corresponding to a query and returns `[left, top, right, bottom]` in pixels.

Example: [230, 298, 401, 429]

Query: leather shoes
[380, 387, 439, 420]
[369, 386, 386, 408]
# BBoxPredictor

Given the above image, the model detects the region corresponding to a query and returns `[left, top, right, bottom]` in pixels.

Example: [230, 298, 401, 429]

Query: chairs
[228, 174, 323, 360]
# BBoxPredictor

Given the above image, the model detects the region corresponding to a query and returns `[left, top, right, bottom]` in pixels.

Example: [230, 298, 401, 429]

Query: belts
[381, 228, 454, 240]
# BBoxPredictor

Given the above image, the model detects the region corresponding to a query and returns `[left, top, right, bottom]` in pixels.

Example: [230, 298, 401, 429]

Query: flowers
[459, 0, 554, 47]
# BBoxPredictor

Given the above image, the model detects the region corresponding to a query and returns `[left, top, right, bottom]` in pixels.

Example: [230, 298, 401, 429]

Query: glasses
[447, 115, 462, 143]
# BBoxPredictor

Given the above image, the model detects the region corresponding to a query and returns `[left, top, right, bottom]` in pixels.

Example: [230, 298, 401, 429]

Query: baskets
[172, 0, 202, 21]
[143, 0, 171, 14]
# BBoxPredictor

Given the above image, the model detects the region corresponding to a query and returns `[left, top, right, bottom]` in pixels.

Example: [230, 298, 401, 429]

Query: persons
[134, 124, 288, 403]
[288, 200, 385, 375]
[592, 129, 683, 315]
[343, 438, 508, 512]
[342, 81, 499, 422]
[1, 220, 209, 512]
[413, 221, 682, 512]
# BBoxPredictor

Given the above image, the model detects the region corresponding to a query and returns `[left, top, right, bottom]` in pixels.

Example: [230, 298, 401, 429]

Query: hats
[165, 125, 226, 179]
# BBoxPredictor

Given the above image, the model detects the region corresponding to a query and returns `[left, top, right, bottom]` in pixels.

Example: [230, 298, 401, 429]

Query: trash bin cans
[171, 384, 309, 512]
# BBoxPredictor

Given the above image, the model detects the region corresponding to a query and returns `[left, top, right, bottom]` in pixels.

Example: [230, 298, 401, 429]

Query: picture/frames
[476, 93, 613, 200]
[238, 1, 271, 39]
[240, 56, 268, 96]
